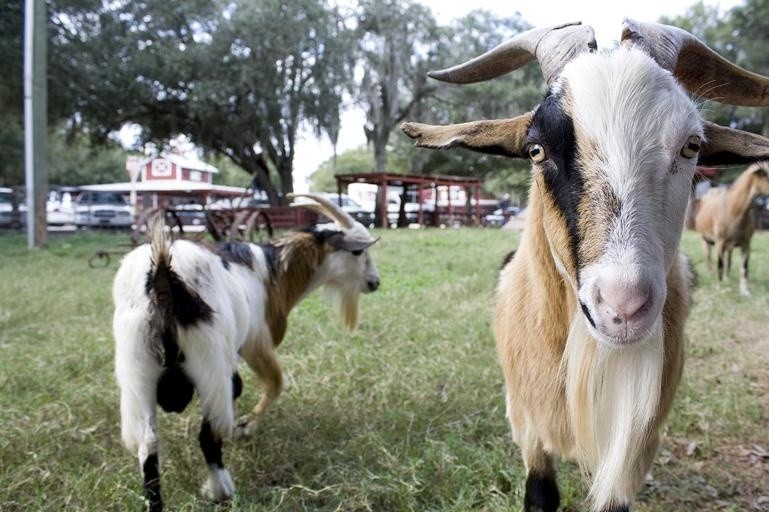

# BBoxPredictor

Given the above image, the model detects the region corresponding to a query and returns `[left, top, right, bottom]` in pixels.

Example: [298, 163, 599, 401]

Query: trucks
[348, 184, 435, 227]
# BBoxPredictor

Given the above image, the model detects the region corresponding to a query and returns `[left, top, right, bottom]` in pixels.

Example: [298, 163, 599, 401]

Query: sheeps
[111, 192, 381, 511]
[695, 162, 768, 298]
[401, 18, 769, 512]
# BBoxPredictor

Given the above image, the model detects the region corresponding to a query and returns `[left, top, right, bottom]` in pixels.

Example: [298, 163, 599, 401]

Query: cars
[166, 205, 208, 225]
[76, 192, 133, 229]
[1, 187, 27, 227]
[314, 193, 370, 228]
